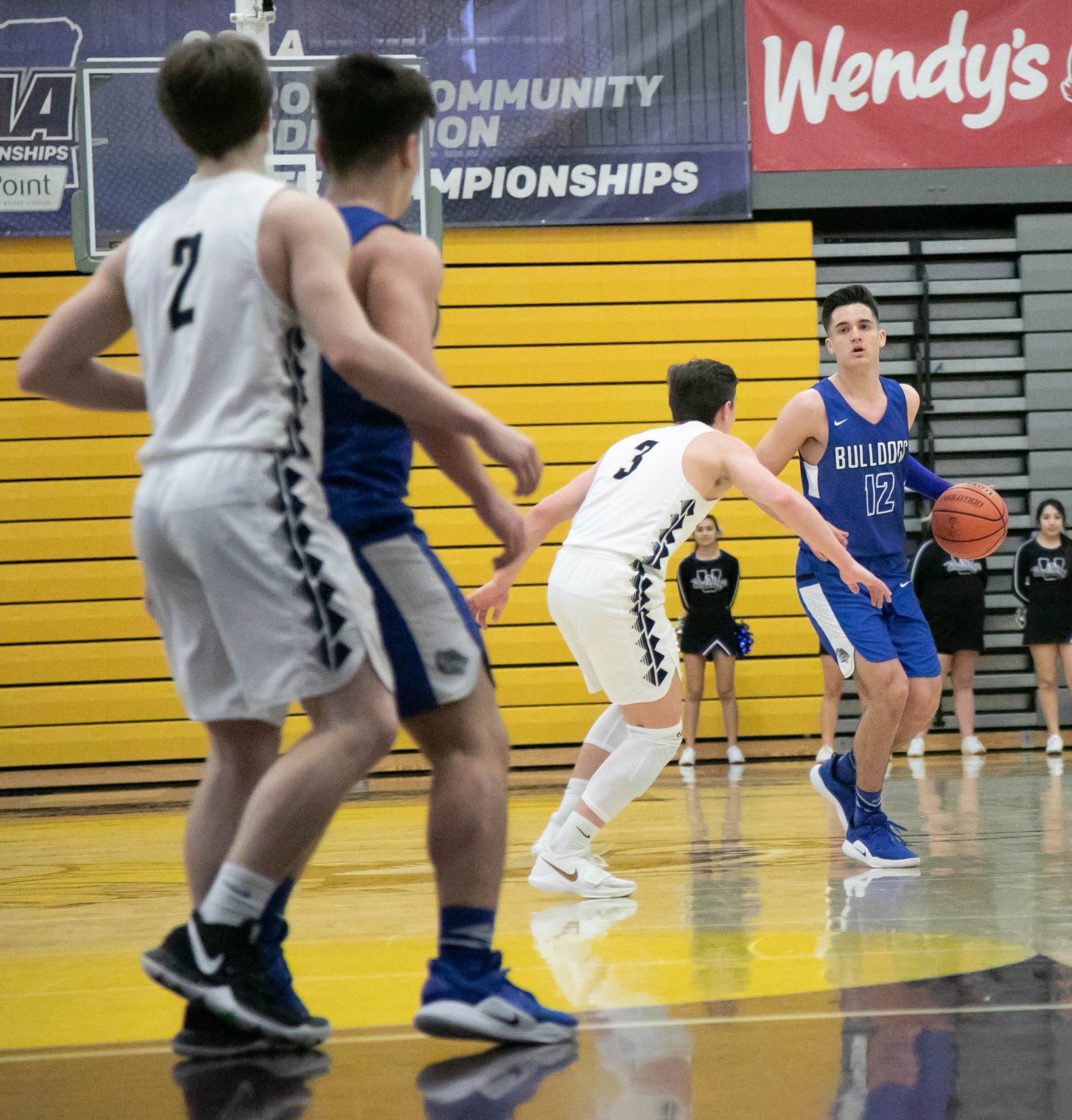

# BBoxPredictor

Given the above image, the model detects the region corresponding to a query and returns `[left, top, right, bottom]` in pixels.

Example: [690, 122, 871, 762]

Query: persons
[675, 498, 1072, 764]
[755, 286, 995, 866]
[18, 33, 540, 1055]
[249, 51, 580, 1045]
[455, 357, 894, 899]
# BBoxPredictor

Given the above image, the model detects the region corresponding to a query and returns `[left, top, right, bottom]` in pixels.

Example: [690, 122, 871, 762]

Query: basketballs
[932, 482, 1009, 560]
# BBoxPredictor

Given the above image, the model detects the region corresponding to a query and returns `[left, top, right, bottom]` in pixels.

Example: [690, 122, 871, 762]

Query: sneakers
[815, 745, 833, 762]
[1044, 755, 1064, 775]
[1046, 735, 1064, 754]
[906, 738, 924, 756]
[416, 1043, 581, 1120]
[809, 752, 857, 832]
[960, 736, 986, 754]
[679, 766, 696, 782]
[170, 1049, 330, 1120]
[842, 813, 922, 868]
[726, 745, 745, 763]
[678, 747, 696, 765]
[530, 812, 568, 854]
[530, 899, 636, 950]
[907, 757, 925, 779]
[140, 907, 330, 1041]
[171, 1001, 272, 1056]
[726, 765, 744, 781]
[844, 869, 921, 903]
[527, 861, 637, 898]
[961, 755, 986, 778]
[415, 949, 577, 1045]
